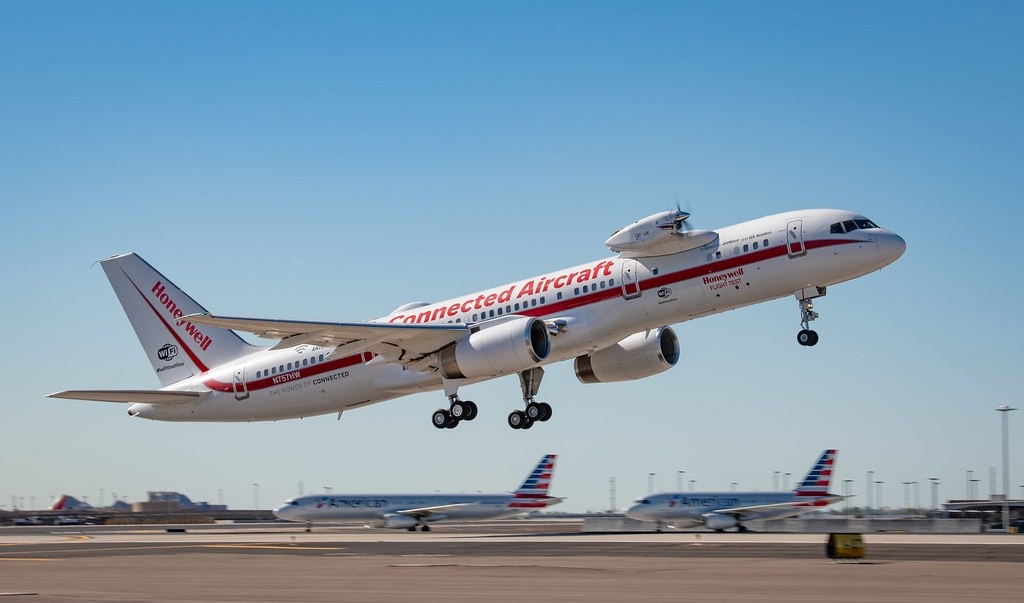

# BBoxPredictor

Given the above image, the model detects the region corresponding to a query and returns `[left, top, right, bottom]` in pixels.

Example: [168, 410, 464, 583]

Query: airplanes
[273, 453, 566, 534]
[46, 210, 907, 430]
[622, 450, 854, 534]
